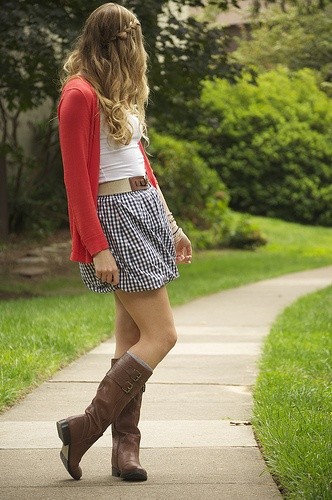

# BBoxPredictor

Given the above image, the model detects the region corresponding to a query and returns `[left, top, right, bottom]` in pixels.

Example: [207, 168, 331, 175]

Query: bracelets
[163, 211, 185, 245]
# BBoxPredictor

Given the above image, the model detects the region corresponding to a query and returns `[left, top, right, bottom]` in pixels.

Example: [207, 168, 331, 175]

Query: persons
[54, 3, 194, 481]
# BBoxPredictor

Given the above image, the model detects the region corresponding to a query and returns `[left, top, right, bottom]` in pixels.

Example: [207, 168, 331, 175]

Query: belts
[98, 174, 153, 197]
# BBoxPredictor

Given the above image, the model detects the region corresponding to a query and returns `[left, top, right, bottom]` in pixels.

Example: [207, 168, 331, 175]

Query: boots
[110, 356, 148, 481]
[57, 351, 153, 481]
